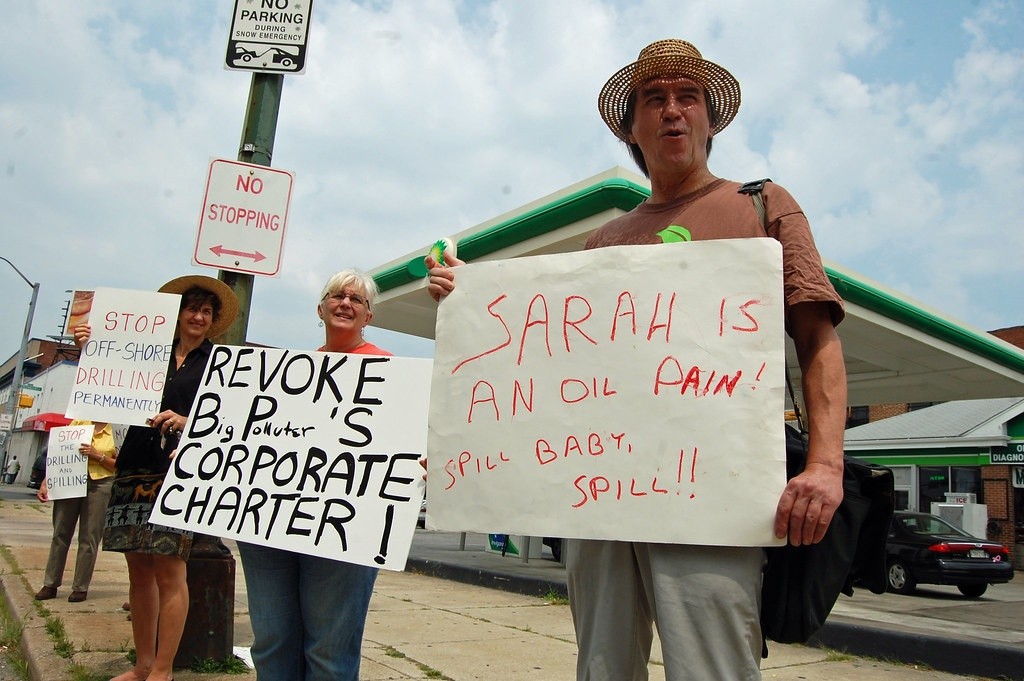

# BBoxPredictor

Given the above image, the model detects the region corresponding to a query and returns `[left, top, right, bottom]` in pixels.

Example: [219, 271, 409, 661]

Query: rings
[85, 450, 87, 452]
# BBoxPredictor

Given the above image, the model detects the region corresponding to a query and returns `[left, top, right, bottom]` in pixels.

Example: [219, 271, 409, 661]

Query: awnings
[22, 414, 73, 432]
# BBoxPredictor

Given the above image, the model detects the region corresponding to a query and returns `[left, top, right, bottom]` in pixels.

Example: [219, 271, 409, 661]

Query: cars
[416, 500, 426, 528]
[882, 510, 1014, 597]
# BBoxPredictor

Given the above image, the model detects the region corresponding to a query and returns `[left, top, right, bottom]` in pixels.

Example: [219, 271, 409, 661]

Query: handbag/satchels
[756, 428, 894, 646]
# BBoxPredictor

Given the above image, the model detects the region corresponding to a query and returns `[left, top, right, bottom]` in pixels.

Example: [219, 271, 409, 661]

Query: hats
[158, 275, 238, 340]
[597, 41, 742, 138]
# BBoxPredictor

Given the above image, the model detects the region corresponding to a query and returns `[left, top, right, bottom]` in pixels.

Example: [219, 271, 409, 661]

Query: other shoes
[68, 590, 87, 602]
[35, 586, 57, 600]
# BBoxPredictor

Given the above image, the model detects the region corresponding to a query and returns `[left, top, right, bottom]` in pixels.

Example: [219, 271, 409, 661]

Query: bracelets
[99, 454, 105, 463]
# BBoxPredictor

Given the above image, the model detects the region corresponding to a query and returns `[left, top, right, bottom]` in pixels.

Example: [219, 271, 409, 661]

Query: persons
[35, 420, 116, 602]
[73, 275, 239, 681]
[423, 39, 847, 681]
[6, 456, 21, 484]
[170, 269, 428, 681]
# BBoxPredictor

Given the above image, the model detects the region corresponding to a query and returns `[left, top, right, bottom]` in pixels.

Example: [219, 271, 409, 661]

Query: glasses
[321, 290, 371, 312]
